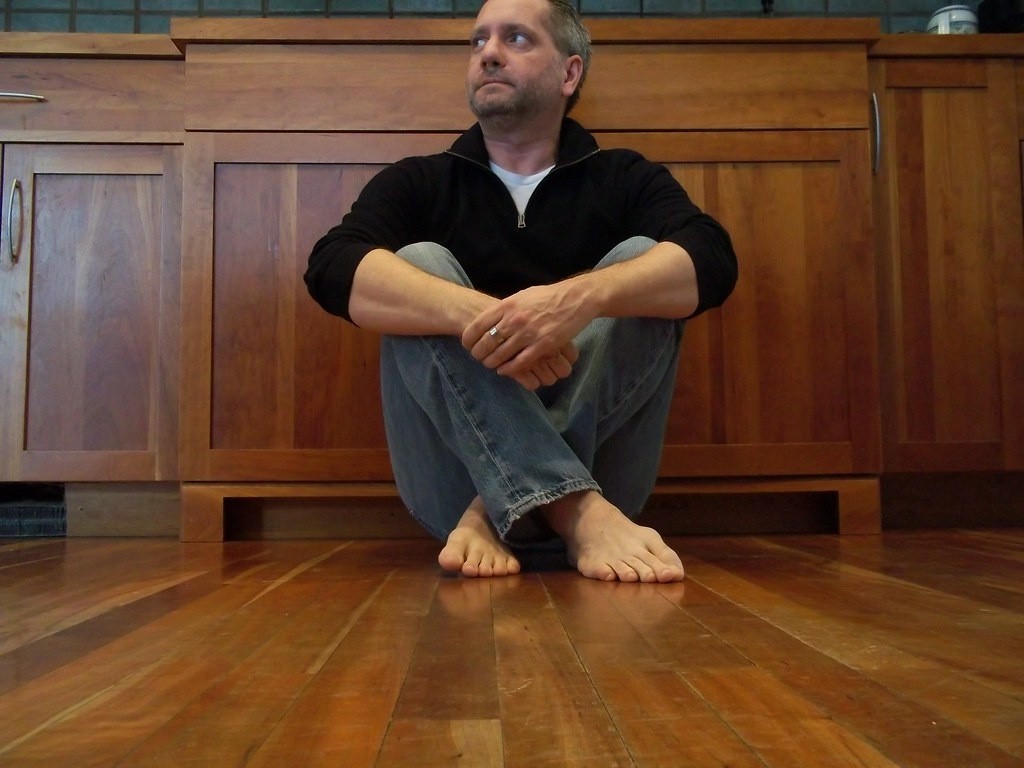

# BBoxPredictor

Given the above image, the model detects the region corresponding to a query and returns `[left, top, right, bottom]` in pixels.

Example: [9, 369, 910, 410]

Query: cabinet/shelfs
[0, 14, 1021, 544]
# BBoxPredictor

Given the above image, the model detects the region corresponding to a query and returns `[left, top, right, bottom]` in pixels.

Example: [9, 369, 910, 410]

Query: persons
[302, 0, 738, 583]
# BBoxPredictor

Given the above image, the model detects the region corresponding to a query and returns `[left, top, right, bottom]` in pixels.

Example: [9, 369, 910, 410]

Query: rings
[489, 326, 505, 345]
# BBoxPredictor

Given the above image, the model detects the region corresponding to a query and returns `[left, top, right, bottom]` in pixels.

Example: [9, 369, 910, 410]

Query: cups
[926, 4, 978, 36]
